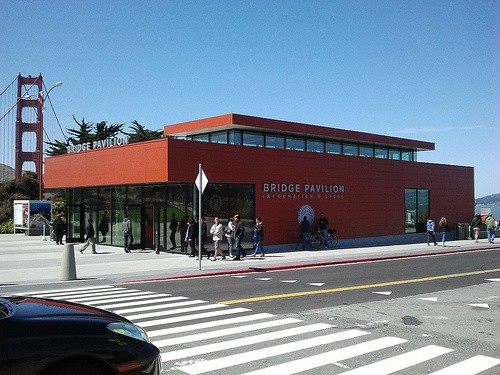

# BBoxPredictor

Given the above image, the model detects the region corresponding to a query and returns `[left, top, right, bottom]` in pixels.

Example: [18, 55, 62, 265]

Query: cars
[0, 294, 163, 375]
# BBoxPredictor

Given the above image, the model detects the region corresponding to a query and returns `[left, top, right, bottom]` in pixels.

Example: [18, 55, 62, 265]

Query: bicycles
[308, 227, 339, 249]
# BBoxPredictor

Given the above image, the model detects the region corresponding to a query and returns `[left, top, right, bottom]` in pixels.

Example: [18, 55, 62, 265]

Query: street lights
[26, 81, 64, 202]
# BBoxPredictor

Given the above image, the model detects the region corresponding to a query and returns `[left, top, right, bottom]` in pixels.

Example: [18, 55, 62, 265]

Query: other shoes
[79, 248, 84, 254]
[488, 240, 494, 244]
[476, 240, 478, 243]
[233, 257, 240, 260]
[196, 256, 202, 260]
[170, 247, 175, 250]
[60, 243, 64, 245]
[434, 243, 438, 246]
[56, 243, 59, 245]
[124, 248, 131, 253]
[189, 254, 195, 257]
[221, 256, 226, 260]
[230, 253, 234, 258]
[211, 256, 216, 261]
[260, 254, 265, 257]
[92, 251, 98, 254]
[240, 253, 246, 258]
[252, 253, 256, 257]
[207, 252, 212, 259]
[428, 244, 430, 246]
[325, 245, 328, 248]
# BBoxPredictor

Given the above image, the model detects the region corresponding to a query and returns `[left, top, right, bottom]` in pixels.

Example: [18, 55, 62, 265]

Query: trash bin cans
[455, 222, 469, 239]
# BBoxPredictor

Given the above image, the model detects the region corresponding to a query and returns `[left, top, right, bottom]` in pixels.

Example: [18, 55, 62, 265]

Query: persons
[52, 215, 65, 245]
[178, 216, 189, 254]
[251, 218, 265, 257]
[79, 218, 98, 254]
[300, 216, 313, 250]
[426, 217, 438, 246]
[99, 214, 108, 242]
[169, 213, 178, 250]
[209, 217, 226, 261]
[317, 212, 329, 249]
[437, 217, 450, 247]
[225, 215, 246, 261]
[470, 214, 482, 243]
[485, 213, 495, 244]
[122, 214, 134, 253]
[185, 216, 212, 260]
